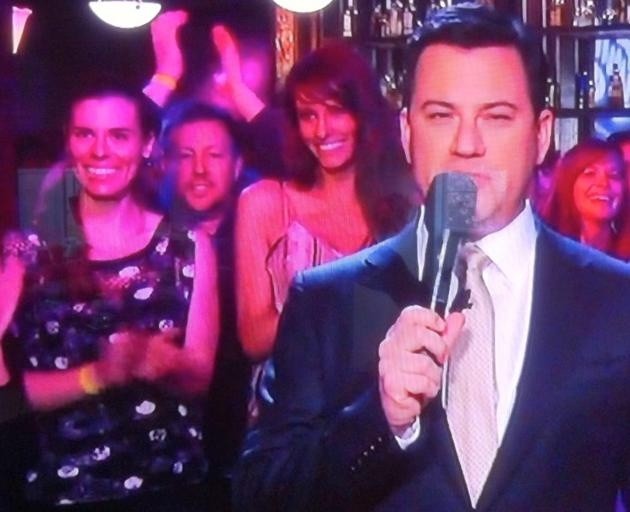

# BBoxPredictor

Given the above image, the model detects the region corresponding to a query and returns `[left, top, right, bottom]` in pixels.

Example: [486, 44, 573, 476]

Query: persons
[230, 4, 629, 512]
[533, 130, 630, 264]
[1, 8, 287, 511]
[233, 37, 424, 431]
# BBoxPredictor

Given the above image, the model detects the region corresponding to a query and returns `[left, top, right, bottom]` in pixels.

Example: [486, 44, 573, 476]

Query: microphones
[417, 172, 478, 364]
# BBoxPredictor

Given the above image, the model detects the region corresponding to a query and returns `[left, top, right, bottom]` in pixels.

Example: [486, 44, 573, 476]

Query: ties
[446, 246, 498, 512]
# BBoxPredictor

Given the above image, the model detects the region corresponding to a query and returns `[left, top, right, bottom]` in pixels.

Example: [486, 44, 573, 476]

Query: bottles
[575, 68, 595, 110]
[342, 0, 360, 37]
[606, 63, 624, 108]
[373, 0, 416, 40]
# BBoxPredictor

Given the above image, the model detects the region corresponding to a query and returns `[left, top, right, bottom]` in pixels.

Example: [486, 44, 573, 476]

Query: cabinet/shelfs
[310, 0, 629, 169]
